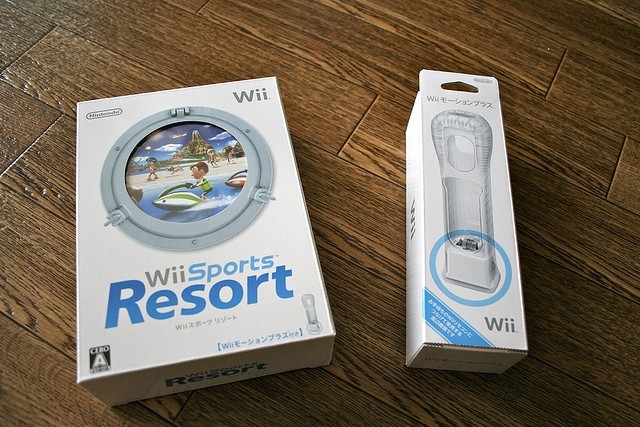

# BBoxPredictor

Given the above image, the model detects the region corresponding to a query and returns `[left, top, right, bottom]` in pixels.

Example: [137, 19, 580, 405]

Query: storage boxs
[75, 77, 337, 410]
[404, 69, 530, 375]
